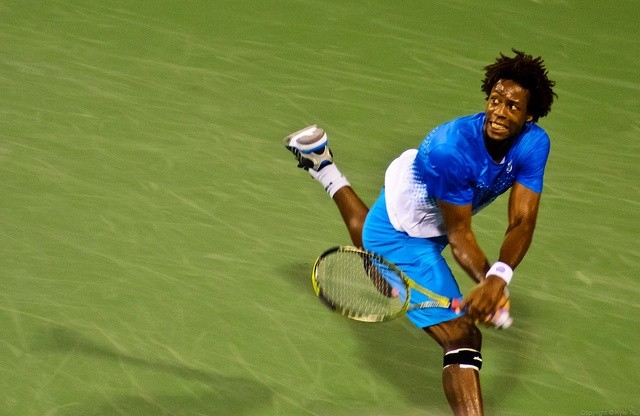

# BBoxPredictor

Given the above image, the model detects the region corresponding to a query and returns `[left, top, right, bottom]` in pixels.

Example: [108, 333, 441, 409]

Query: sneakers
[283, 124, 333, 171]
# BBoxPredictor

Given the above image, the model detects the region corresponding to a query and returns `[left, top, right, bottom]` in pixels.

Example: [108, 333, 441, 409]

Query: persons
[282, 46, 560, 416]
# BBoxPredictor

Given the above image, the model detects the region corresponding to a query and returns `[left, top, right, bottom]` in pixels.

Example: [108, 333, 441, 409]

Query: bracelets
[485, 260, 515, 286]
